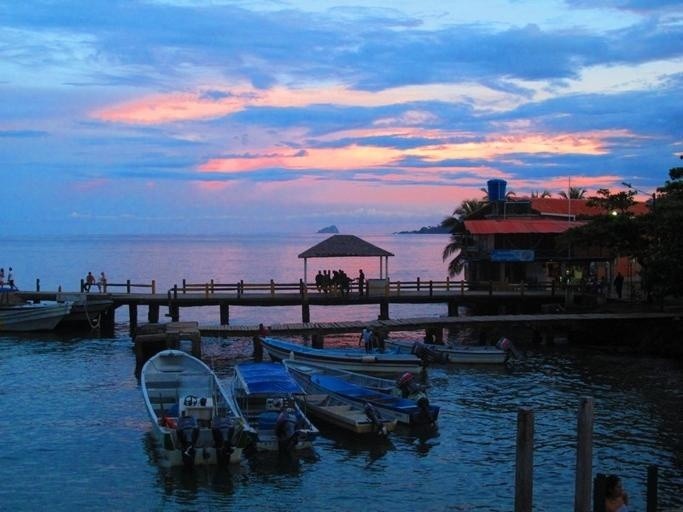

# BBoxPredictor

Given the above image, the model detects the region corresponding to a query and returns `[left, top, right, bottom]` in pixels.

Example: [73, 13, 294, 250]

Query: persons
[314, 267, 354, 297]
[96, 272, 107, 293]
[82, 271, 96, 294]
[0, 267, 4, 289]
[612, 271, 625, 300]
[6, 266, 18, 291]
[604, 474, 630, 511]
[356, 269, 364, 296]
[358, 326, 374, 353]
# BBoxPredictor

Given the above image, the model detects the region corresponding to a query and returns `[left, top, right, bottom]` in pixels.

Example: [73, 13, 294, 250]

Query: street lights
[620, 181, 655, 214]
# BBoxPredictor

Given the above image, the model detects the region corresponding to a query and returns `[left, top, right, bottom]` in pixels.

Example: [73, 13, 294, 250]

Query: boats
[0, 292, 113, 339]
